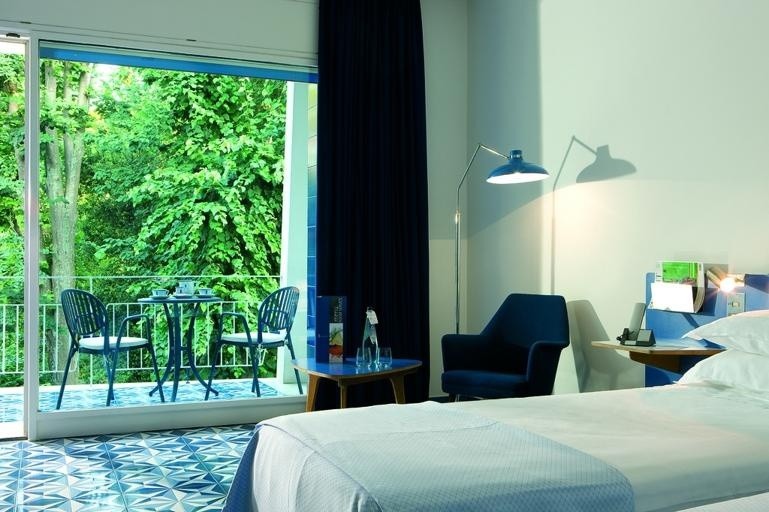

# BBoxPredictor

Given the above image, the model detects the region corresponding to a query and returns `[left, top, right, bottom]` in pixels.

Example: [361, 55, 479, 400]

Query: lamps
[456, 143, 549, 333]
[706, 266, 744, 293]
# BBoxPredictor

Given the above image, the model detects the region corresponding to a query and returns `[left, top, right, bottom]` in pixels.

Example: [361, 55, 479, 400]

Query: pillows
[681, 310, 769, 358]
[673, 349, 769, 400]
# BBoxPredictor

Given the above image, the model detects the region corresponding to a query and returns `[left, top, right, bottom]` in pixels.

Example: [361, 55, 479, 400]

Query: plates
[195, 294, 215, 298]
[172, 295, 194, 299]
[149, 296, 170, 299]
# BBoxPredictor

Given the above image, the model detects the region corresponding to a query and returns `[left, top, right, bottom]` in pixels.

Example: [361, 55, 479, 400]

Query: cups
[375, 348, 393, 368]
[179, 280, 194, 294]
[198, 288, 211, 294]
[175, 287, 185, 294]
[153, 289, 169, 296]
[356, 347, 372, 368]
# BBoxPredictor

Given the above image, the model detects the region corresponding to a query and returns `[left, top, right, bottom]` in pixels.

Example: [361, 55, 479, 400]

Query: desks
[138, 297, 221, 403]
[591, 274, 727, 388]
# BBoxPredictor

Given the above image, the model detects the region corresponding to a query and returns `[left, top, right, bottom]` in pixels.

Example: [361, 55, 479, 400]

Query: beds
[222, 384, 769, 512]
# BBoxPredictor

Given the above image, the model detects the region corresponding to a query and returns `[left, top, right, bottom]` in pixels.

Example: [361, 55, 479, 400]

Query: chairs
[57, 288, 164, 409]
[205, 287, 303, 401]
[441, 293, 569, 402]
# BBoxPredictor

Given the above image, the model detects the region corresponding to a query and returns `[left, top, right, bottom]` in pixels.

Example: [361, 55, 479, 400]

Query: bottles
[361, 307, 379, 366]
